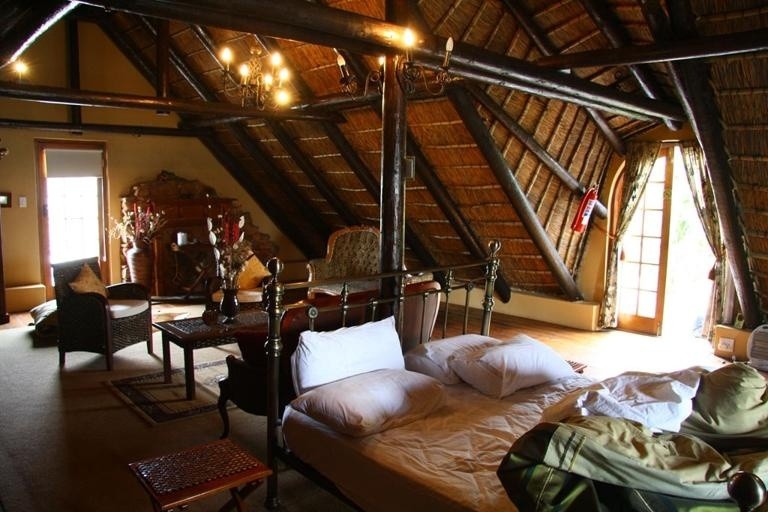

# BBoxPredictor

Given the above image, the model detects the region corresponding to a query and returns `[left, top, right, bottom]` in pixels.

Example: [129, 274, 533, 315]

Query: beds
[263, 239, 768, 512]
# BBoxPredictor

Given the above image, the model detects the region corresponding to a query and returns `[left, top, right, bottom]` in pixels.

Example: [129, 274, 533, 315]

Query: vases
[219, 287, 240, 324]
[126, 241, 148, 287]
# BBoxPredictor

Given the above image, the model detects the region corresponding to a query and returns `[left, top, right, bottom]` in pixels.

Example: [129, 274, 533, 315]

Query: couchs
[219, 280, 442, 438]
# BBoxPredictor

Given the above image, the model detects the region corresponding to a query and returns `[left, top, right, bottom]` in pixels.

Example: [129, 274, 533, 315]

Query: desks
[172, 243, 217, 301]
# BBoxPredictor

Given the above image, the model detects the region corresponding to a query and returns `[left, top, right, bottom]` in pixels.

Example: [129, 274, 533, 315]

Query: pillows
[291, 315, 572, 438]
[239, 250, 271, 289]
[67, 261, 111, 299]
[235, 331, 268, 362]
[30, 299, 57, 336]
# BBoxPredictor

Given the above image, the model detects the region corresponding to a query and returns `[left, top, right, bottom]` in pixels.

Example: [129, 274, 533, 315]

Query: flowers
[207, 210, 256, 288]
[105, 198, 168, 245]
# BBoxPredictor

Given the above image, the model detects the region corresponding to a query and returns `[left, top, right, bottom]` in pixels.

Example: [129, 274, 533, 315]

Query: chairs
[50, 256, 153, 371]
[306, 225, 380, 300]
[747, 324, 768, 372]
[203, 224, 280, 309]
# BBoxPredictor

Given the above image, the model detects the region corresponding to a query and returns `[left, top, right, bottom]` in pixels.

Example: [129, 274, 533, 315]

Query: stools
[128, 437, 273, 512]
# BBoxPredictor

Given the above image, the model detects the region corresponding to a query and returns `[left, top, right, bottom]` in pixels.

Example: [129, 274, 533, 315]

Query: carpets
[0, 305, 357, 512]
[102, 356, 243, 427]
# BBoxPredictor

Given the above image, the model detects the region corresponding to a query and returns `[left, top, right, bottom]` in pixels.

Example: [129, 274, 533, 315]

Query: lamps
[220, 38, 290, 111]
[336, 28, 463, 102]
